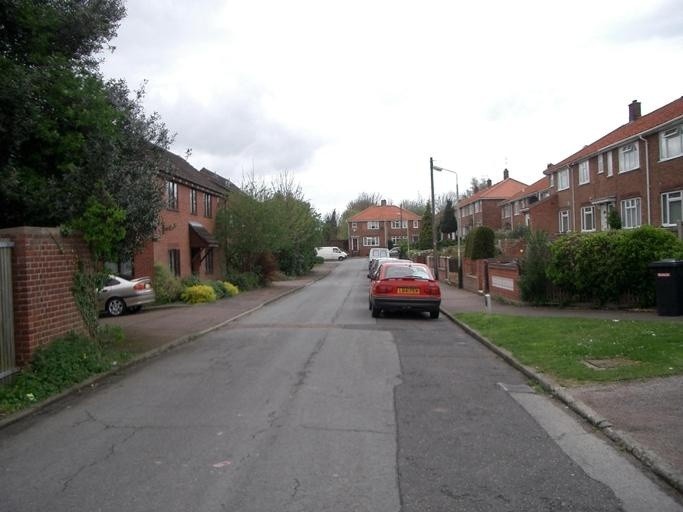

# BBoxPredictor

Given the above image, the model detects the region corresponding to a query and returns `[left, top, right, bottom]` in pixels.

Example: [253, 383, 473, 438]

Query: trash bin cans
[649, 258, 682, 316]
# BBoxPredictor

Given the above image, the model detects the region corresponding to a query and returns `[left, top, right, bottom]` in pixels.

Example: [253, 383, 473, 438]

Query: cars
[389, 247, 401, 256]
[96, 273, 156, 316]
[368, 257, 441, 319]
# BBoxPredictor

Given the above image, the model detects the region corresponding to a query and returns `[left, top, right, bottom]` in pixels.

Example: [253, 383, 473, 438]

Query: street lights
[434, 166, 463, 289]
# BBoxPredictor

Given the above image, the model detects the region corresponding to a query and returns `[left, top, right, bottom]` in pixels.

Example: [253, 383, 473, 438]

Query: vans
[367, 248, 390, 263]
[314, 246, 347, 260]
